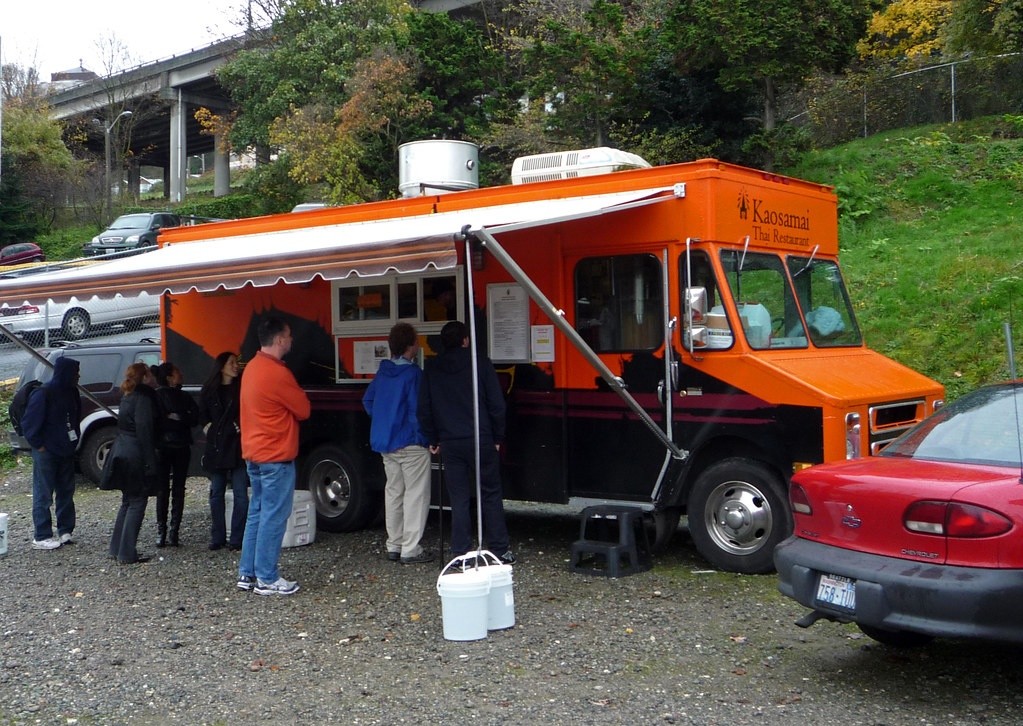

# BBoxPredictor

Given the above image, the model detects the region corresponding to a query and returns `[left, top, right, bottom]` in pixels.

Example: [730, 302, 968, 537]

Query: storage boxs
[704, 314, 749, 348]
[225, 485, 316, 547]
[0, 513, 8, 555]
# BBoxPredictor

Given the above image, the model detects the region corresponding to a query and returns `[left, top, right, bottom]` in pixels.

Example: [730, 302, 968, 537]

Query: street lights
[104, 111, 132, 228]
[194, 154, 205, 173]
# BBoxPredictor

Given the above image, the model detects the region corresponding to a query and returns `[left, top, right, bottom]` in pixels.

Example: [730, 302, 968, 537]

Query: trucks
[0, 140, 945, 574]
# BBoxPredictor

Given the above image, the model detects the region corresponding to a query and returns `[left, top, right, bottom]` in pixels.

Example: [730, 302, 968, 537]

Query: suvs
[82, 213, 181, 261]
[7, 337, 162, 487]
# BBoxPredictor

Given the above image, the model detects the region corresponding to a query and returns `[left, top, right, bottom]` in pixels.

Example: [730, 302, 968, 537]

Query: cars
[0, 243, 46, 266]
[0, 291, 161, 342]
[773, 378, 1023, 663]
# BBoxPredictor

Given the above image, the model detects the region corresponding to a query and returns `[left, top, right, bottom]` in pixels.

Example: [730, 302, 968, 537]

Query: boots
[155, 519, 167, 546]
[169, 521, 179, 547]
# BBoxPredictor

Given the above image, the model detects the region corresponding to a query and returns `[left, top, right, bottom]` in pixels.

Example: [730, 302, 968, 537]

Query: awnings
[1, 183, 684, 455]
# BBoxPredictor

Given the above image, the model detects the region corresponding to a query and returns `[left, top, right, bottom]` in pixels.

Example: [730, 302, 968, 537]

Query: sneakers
[31, 539, 62, 550]
[237, 576, 255, 590]
[253, 577, 301, 596]
[59, 533, 72, 544]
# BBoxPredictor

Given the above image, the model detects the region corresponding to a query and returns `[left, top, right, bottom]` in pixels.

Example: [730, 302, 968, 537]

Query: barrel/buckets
[0, 513, 9, 554]
[462, 549, 515, 631]
[435, 554, 492, 641]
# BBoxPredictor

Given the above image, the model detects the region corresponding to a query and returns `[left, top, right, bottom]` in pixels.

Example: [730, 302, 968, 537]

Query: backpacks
[9, 380, 81, 437]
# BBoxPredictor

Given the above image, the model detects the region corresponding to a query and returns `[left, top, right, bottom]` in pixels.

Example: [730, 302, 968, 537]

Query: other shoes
[388, 552, 400, 560]
[229, 544, 242, 551]
[208, 540, 227, 550]
[492, 550, 517, 564]
[449, 558, 471, 572]
[399, 550, 435, 563]
[136, 553, 149, 562]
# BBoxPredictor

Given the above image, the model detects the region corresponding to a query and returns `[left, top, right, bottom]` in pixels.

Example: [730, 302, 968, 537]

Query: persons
[202, 352, 242, 549]
[235, 317, 311, 598]
[22, 356, 82, 550]
[361, 322, 439, 563]
[97, 364, 164, 564]
[149, 362, 199, 547]
[423, 319, 515, 567]
